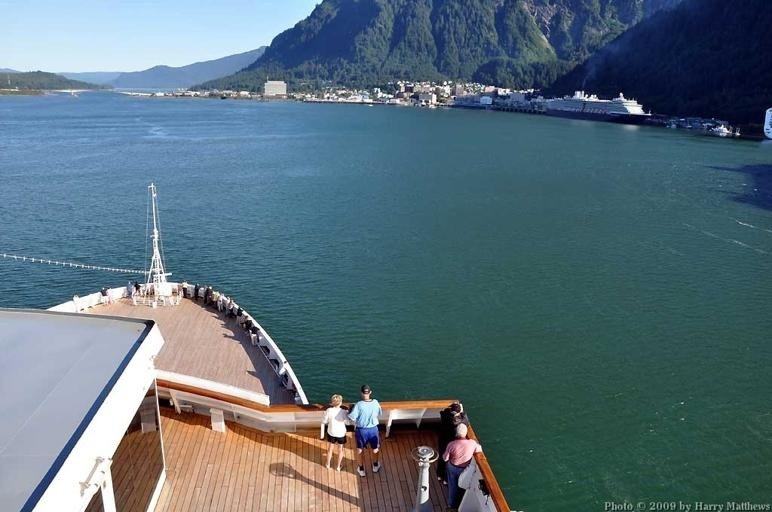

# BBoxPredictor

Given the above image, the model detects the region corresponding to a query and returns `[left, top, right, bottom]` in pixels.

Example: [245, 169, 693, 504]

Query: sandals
[337, 464, 343, 471]
[325, 463, 330, 468]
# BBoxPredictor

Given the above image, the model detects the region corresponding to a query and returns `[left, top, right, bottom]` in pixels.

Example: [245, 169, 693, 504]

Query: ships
[545, 89, 653, 124]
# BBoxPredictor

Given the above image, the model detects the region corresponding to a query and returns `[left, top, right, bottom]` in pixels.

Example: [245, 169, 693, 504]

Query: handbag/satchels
[453, 413, 468, 426]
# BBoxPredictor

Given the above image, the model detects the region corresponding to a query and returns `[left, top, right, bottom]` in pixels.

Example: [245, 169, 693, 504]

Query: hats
[361, 384, 370, 394]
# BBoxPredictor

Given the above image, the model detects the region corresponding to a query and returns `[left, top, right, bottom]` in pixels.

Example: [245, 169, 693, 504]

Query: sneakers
[357, 466, 366, 477]
[373, 462, 380, 473]
[437, 477, 448, 484]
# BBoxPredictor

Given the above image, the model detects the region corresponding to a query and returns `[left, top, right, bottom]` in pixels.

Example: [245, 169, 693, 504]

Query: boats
[220, 93, 227, 99]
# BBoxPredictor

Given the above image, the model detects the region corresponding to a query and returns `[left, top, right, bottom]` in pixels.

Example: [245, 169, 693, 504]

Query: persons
[437, 404, 482, 508]
[194, 283, 201, 300]
[181, 280, 188, 298]
[204, 286, 234, 318]
[346, 384, 383, 477]
[73, 294, 80, 312]
[100, 287, 112, 306]
[322, 394, 349, 472]
[236, 307, 259, 345]
[127, 281, 140, 298]
